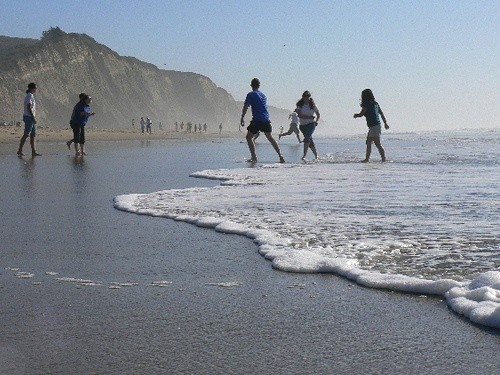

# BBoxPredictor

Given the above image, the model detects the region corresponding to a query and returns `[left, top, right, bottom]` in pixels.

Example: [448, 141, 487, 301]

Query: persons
[240, 79, 286, 163]
[218, 123, 222, 133]
[139, 117, 146, 134]
[239, 123, 241, 132]
[176, 121, 207, 133]
[159, 122, 162, 130]
[279, 108, 303, 143]
[66, 93, 96, 156]
[145, 117, 152, 134]
[17, 82, 43, 156]
[352, 89, 390, 163]
[279, 125, 283, 134]
[132, 119, 135, 128]
[295, 90, 320, 159]
